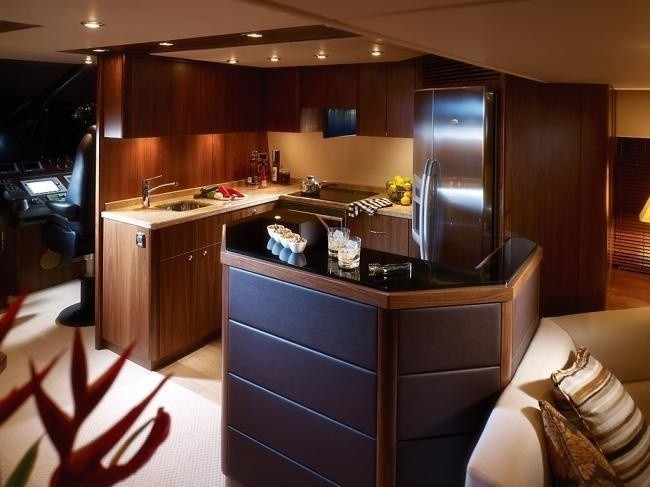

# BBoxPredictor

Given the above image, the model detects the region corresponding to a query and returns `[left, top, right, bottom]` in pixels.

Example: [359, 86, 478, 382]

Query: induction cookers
[288, 188, 380, 203]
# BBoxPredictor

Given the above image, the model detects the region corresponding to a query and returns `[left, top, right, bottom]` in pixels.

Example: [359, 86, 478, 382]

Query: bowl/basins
[266, 238, 308, 267]
[267, 225, 307, 253]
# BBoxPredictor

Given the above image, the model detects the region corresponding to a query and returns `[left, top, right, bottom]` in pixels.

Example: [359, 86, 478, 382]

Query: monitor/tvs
[20, 176, 67, 196]
[63, 175, 71, 184]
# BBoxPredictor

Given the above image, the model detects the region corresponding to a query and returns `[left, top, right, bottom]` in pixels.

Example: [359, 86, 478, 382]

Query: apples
[386, 175, 412, 205]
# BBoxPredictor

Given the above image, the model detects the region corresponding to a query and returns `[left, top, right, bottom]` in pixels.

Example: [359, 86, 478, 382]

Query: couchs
[465, 306, 650, 487]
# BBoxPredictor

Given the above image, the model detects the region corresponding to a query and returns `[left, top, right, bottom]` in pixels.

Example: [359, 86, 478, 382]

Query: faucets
[141, 173, 178, 208]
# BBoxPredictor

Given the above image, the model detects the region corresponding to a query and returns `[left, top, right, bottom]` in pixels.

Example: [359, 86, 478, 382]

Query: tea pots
[299, 176, 322, 196]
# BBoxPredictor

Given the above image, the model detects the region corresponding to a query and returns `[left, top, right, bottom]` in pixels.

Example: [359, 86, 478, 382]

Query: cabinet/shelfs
[232, 201, 277, 222]
[346, 212, 409, 257]
[502, 81, 616, 317]
[102, 201, 232, 372]
[101, 52, 357, 140]
[357, 61, 425, 138]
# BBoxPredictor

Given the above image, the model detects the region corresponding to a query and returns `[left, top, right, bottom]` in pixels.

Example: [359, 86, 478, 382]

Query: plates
[219, 194, 248, 200]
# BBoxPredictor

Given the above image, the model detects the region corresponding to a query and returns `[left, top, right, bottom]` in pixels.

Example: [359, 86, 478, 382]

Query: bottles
[278, 169, 290, 185]
[272, 151, 280, 184]
[260, 166, 267, 188]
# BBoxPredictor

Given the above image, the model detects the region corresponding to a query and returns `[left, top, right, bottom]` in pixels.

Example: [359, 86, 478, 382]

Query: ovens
[279, 202, 347, 240]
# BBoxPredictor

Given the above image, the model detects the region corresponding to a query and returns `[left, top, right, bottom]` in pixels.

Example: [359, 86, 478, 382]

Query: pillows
[538, 400, 616, 487]
[551, 345, 650, 487]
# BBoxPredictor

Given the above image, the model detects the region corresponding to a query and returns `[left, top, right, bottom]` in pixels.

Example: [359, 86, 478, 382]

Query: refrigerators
[410, 86, 498, 270]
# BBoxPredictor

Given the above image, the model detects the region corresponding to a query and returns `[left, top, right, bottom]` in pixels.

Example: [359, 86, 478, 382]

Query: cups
[327, 227, 350, 258]
[328, 259, 339, 277]
[338, 269, 360, 282]
[338, 236, 361, 269]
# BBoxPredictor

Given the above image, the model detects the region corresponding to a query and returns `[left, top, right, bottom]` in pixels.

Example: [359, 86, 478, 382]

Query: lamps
[638, 196, 650, 223]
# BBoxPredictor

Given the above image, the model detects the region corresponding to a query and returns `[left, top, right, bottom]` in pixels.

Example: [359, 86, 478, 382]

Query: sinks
[155, 198, 214, 212]
